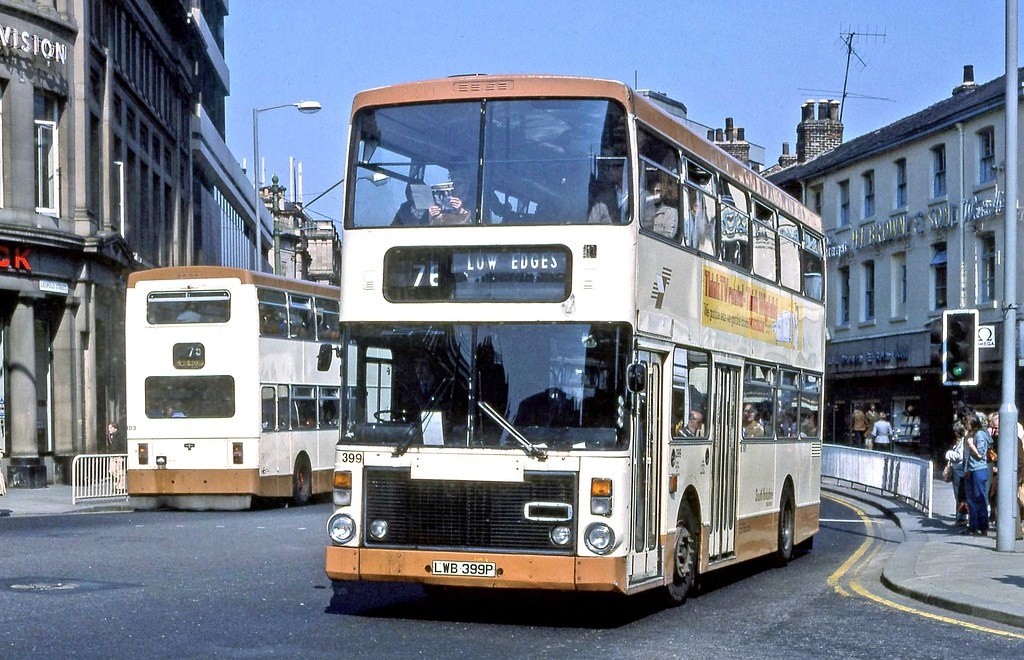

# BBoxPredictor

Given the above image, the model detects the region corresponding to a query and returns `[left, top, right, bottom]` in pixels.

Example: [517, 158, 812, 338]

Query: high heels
[961, 528, 988, 536]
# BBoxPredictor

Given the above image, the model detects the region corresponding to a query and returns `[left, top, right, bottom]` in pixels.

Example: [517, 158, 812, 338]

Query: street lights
[255, 100, 322, 272]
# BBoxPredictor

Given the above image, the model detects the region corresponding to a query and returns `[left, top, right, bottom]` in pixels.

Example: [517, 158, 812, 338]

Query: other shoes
[952, 519, 967, 527]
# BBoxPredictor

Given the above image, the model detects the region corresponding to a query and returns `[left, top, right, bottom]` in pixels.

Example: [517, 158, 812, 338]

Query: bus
[126, 265, 395, 507]
[324, 73, 842, 617]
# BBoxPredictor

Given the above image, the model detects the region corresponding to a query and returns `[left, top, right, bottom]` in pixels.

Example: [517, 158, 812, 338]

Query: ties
[424, 383, 428, 394]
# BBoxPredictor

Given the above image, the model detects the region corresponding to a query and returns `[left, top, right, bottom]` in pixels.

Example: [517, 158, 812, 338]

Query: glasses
[800, 413, 808, 418]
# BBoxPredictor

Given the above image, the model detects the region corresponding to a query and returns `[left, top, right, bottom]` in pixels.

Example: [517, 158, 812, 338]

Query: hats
[477, 344, 494, 359]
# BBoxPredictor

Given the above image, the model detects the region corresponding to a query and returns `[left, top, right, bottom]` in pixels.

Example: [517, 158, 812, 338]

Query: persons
[391, 166, 817, 438]
[852, 403, 1024, 539]
[147, 302, 342, 429]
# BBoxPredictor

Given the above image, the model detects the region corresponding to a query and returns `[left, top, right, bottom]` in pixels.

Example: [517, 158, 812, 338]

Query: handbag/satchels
[942, 459, 953, 482]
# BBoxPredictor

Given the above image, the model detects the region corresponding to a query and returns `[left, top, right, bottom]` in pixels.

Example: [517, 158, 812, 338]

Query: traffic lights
[941, 309, 980, 386]
